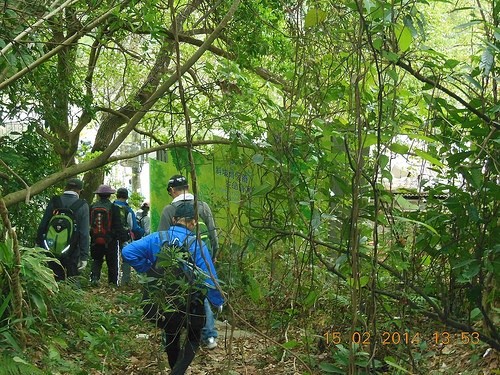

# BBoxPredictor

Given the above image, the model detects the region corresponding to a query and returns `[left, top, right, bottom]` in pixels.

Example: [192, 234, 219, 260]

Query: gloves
[218, 305, 224, 314]
[77, 260, 88, 271]
[34, 243, 40, 248]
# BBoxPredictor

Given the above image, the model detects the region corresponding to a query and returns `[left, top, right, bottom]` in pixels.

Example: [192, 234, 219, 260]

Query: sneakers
[201, 336, 217, 350]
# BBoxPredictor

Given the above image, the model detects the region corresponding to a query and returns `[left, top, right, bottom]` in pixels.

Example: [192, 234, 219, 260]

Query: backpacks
[89, 203, 113, 254]
[117, 204, 130, 229]
[140, 231, 197, 334]
[45, 196, 86, 261]
[131, 215, 147, 239]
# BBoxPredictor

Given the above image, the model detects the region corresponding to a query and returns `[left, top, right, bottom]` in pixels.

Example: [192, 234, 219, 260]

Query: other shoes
[90, 276, 99, 288]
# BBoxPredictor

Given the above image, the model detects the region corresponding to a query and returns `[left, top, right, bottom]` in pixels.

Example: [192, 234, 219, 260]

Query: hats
[174, 202, 195, 219]
[92, 184, 116, 194]
[141, 203, 149, 209]
[65, 179, 83, 191]
[115, 188, 129, 197]
[167, 175, 188, 190]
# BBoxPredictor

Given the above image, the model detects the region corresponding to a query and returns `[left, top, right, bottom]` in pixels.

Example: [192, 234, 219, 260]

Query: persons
[89, 184, 122, 286]
[121, 203, 224, 375]
[157, 174, 221, 350]
[36, 178, 89, 282]
[134, 202, 151, 237]
[112, 188, 144, 289]
